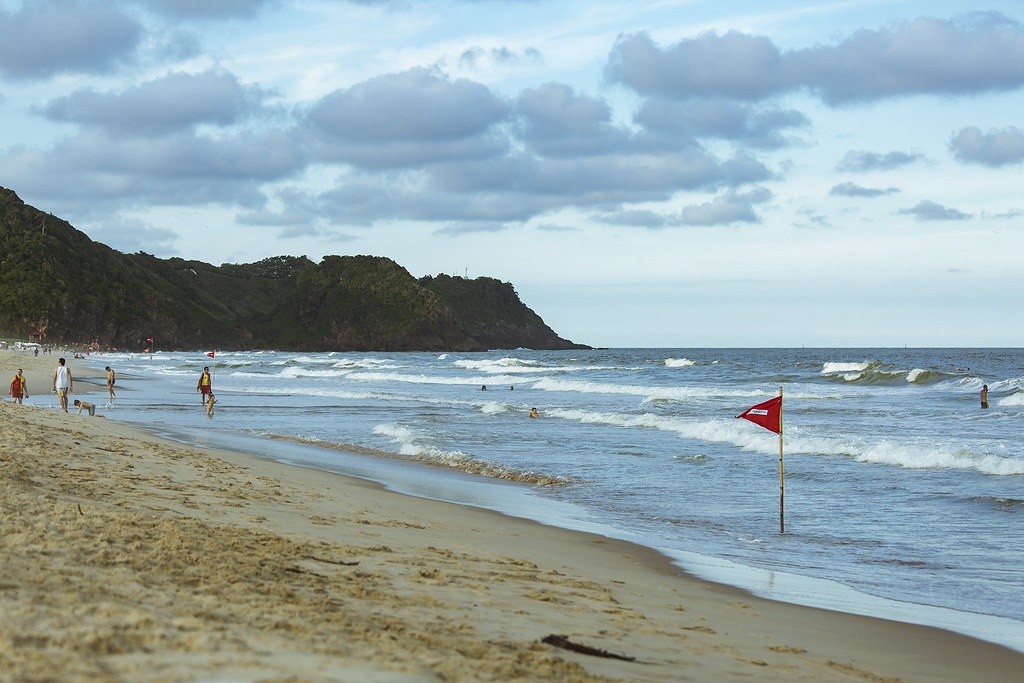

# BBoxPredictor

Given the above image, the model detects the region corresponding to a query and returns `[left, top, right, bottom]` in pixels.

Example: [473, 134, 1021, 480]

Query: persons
[105, 366, 115, 397]
[34, 347, 38, 356]
[529, 408, 539, 417]
[52, 358, 73, 413]
[43, 346, 51, 356]
[481, 385, 486, 391]
[150, 355, 152, 361]
[206, 395, 218, 415]
[8, 368, 29, 404]
[980, 384, 989, 408]
[510, 386, 513, 390]
[74, 400, 105, 418]
[196, 367, 212, 405]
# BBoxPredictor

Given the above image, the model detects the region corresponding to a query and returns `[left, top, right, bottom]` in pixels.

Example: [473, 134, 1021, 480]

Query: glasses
[205, 369, 209, 370]
